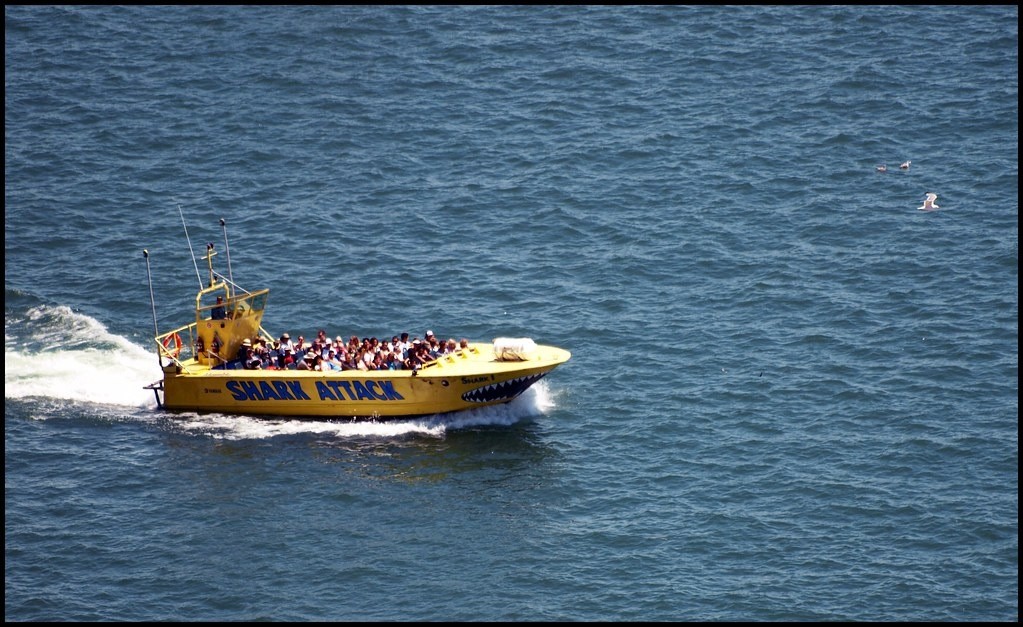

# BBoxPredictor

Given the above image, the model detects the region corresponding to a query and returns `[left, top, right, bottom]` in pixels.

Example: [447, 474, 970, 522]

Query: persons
[237, 328, 467, 371]
[210, 296, 226, 320]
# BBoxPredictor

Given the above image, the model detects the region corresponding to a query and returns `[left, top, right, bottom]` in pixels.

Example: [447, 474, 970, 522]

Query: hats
[325, 338, 332, 345]
[427, 331, 433, 336]
[283, 333, 291, 339]
[258, 337, 266, 342]
[304, 352, 317, 359]
[217, 296, 222, 301]
[242, 338, 251, 346]
[412, 339, 421, 344]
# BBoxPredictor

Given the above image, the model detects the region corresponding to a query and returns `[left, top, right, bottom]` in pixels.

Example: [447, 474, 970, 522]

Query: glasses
[335, 340, 341, 343]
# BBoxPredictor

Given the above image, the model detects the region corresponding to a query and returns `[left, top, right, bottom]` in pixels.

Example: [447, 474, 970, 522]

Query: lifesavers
[163, 334, 181, 359]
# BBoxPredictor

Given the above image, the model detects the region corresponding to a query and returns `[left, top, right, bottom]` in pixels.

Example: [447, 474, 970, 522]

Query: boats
[142, 204, 571, 420]
